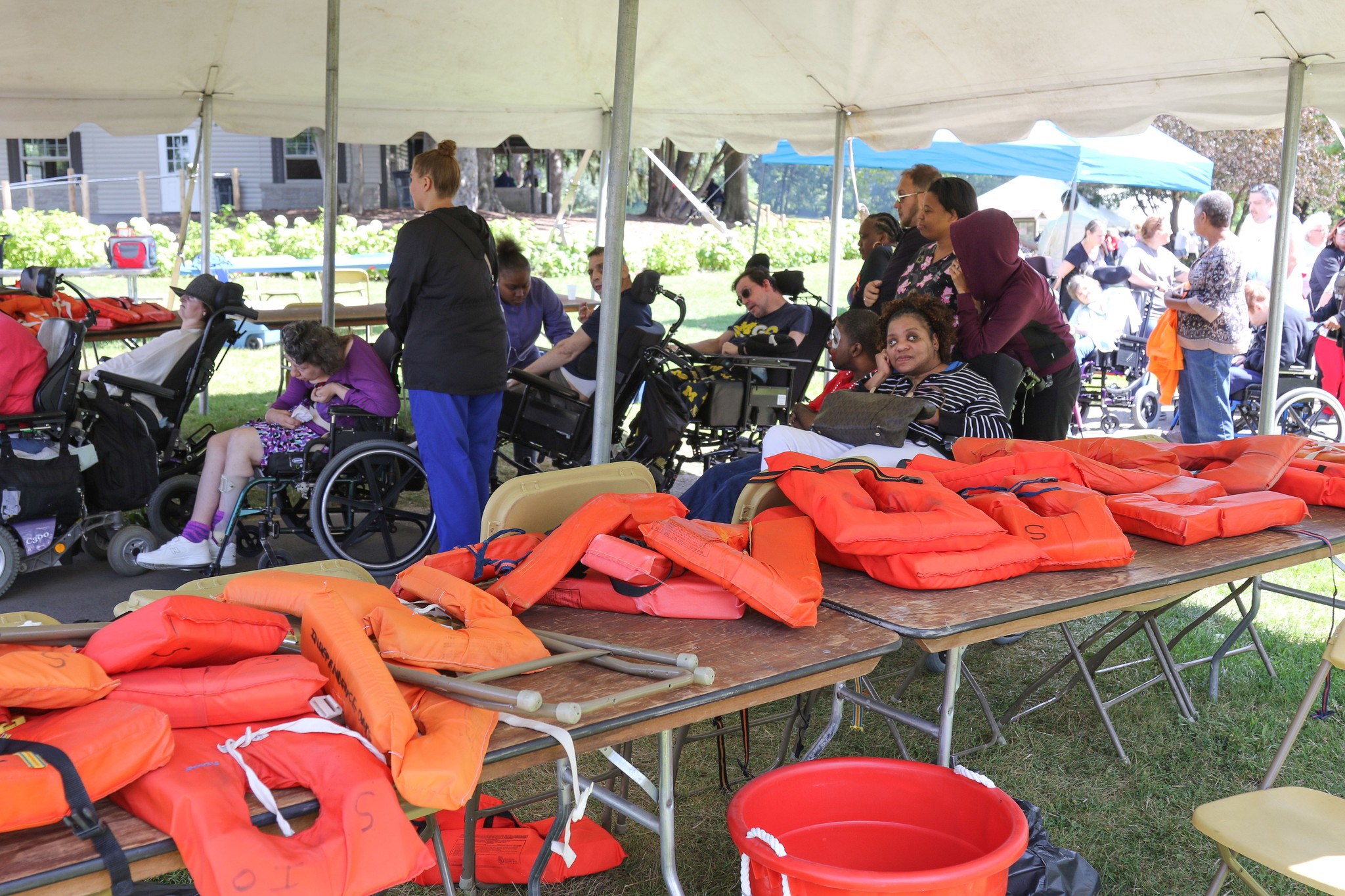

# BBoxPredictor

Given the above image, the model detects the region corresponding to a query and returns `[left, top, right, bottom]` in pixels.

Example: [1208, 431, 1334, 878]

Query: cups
[568, 285, 576, 301]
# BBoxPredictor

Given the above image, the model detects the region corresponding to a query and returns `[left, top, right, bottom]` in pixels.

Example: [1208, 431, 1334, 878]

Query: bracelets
[265, 408, 275, 424]
[1168, 288, 1175, 298]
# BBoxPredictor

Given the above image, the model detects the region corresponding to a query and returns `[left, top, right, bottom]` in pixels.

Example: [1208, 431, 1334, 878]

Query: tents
[754, 120, 1214, 260]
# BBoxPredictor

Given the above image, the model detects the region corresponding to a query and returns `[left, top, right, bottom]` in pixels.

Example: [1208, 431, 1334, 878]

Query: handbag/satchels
[809, 374, 946, 448]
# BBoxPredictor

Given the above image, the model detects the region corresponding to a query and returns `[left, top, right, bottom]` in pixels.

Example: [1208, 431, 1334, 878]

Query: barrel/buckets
[727, 757, 1029, 896]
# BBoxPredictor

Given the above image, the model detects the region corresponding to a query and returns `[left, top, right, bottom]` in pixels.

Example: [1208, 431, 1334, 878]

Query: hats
[169, 274, 224, 316]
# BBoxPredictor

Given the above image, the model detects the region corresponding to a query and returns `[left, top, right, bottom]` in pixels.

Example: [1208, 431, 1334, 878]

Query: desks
[0, 601, 901, 896]
[818, 502, 1345, 771]
[0, 252, 392, 416]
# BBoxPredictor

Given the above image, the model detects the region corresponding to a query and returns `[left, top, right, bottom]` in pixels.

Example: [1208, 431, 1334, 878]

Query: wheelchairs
[1230, 320, 1344, 445]
[1072, 265, 1162, 434]
[654, 254, 835, 493]
[0, 266, 250, 598]
[207, 326, 443, 576]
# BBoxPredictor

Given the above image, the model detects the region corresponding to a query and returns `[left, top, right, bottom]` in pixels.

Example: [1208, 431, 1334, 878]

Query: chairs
[317, 268, 371, 344]
[0, 455, 881, 650]
[1190, 616, 1345, 896]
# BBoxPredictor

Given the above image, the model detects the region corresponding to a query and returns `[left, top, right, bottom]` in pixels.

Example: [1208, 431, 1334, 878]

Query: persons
[1053, 183, 1345, 446]
[495, 169, 515, 188]
[815, 160, 1082, 441]
[702, 178, 725, 211]
[387, 141, 512, 552]
[676, 291, 1013, 522]
[521, 159, 541, 189]
[492, 231, 655, 477]
[79, 272, 225, 427]
[0, 307, 50, 435]
[136, 318, 401, 569]
[615, 269, 814, 493]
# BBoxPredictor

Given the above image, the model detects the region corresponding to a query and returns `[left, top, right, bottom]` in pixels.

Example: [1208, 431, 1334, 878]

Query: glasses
[1250, 184, 1275, 201]
[1153, 216, 1165, 231]
[1336, 226, 1345, 235]
[895, 191, 925, 202]
[1312, 228, 1330, 233]
[736, 281, 762, 306]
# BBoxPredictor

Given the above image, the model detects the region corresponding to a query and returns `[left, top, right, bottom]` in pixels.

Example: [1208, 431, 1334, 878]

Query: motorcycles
[488, 270, 686, 496]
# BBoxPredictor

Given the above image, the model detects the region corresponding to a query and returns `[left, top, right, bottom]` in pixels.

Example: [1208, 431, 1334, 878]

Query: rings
[863, 297, 867, 301]
[1331, 327, 1335, 332]
[956, 264, 960, 269]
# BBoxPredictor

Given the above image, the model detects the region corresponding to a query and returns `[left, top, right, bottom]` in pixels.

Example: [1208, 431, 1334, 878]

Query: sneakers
[207, 531, 237, 566]
[136, 536, 212, 570]
